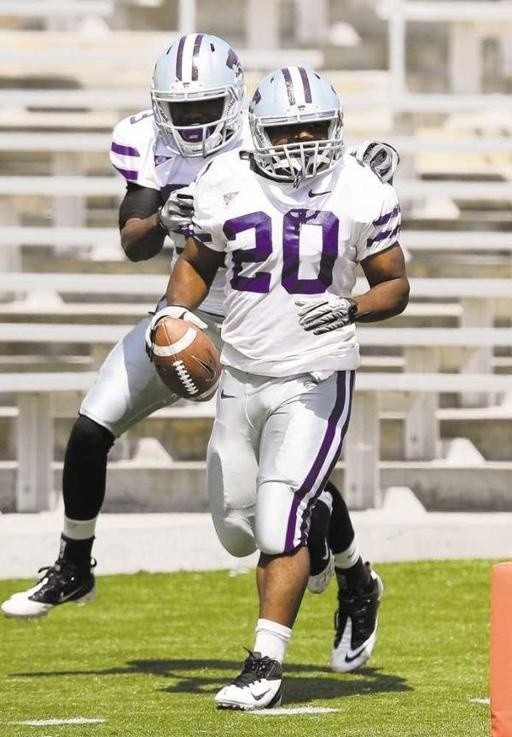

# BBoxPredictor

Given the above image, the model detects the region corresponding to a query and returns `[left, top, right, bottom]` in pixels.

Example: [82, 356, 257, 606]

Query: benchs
[1, 0, 510, 512]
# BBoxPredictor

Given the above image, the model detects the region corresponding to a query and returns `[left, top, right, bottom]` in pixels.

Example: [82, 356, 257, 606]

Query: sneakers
[331, 562, 383, 673]
[1, 557, 96, 617]
[215, 647, 284, 710]
[308, 491, 335, 593]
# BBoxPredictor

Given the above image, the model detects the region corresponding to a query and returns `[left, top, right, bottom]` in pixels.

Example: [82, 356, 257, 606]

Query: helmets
[248, 68, 345, 181]
[151, 33, 245, 158]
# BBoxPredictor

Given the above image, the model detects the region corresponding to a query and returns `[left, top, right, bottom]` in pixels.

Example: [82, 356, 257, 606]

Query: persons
[137, 62, 413, 713]
[2, 27, 403, 673]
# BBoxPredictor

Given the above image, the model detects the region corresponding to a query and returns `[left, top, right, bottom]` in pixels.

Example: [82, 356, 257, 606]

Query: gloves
[145, 306, 209, 362]
[158, 186, 194, 231]
[350, 143, 399, 185]
[295, 298, 358, 334]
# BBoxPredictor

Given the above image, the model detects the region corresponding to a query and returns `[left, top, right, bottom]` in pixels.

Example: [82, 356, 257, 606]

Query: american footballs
[150, 317, 222, 402]
